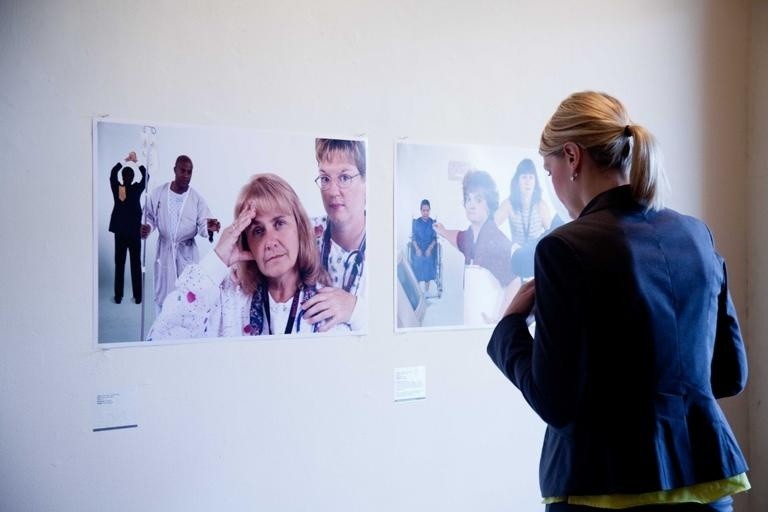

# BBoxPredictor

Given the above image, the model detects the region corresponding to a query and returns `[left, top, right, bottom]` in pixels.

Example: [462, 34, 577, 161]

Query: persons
[107, 151, 152, 305]
[492, 159, 566, 286]
[430, 168, 521, 327]
[408, 198, 441, 301]
[485, 88, 755, 512]
[136, 155, 224, 313]
[228, 137, 364, 338]
[138, 172, 355, 341]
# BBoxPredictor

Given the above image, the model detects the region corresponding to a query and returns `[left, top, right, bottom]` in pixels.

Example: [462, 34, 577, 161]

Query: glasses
[315, 173, 361, 191]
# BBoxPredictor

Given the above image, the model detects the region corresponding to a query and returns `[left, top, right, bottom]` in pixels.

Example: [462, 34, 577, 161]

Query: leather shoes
[114, 297, 141, 304]
